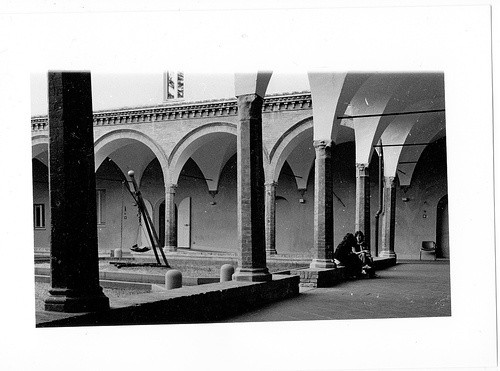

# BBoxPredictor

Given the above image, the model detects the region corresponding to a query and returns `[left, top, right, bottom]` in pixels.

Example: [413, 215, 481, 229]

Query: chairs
[420, 240, 437, 260]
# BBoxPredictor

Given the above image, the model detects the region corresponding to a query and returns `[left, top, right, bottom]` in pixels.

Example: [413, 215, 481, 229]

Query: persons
[333, 232, 370, 278]
[352, 230, 380, 278]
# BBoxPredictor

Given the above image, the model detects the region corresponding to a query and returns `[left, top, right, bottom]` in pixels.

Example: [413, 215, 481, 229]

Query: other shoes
[370, 273, 379, 279]
[362, 264, 371, 275]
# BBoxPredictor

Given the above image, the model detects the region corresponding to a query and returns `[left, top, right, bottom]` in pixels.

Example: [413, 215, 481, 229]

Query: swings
[130, 224, 151, 253]
